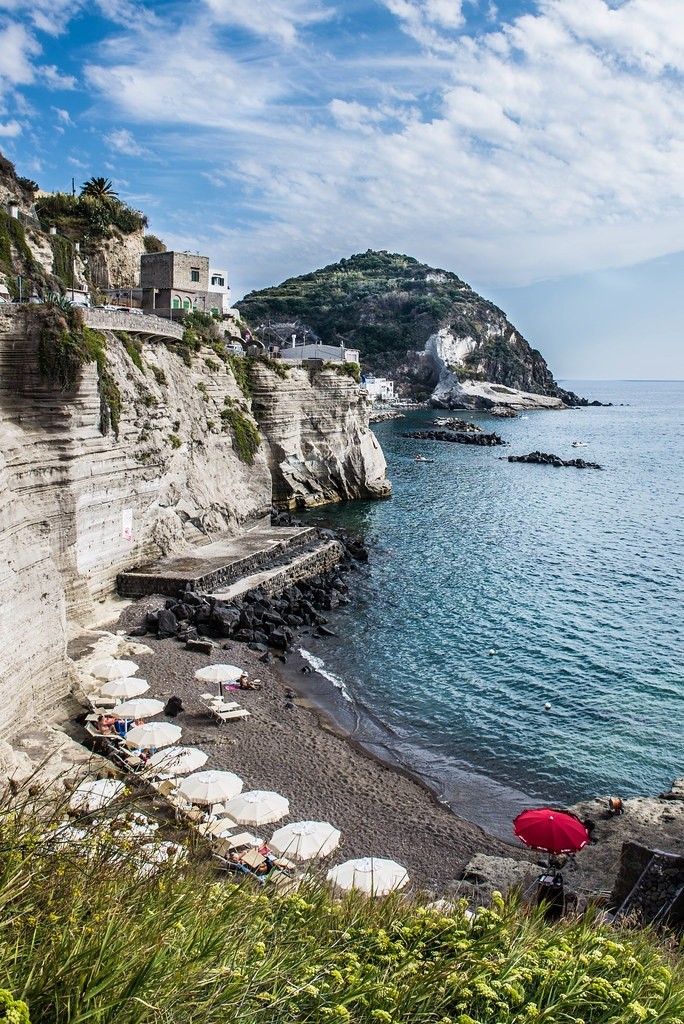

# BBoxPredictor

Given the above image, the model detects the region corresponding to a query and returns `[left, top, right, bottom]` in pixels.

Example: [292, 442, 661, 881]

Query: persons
[226, 851, 287, 875]
[98, 716, 121, 735]
[140, 748, 154, 762]
[240, 672, 261, 690]
[127, 744, 137, 752]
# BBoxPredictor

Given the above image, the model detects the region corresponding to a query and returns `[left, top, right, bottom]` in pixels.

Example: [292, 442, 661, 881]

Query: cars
[116, 308, 144, 314]
[90, 305, 116, 311]
[68, 301, 87, 307]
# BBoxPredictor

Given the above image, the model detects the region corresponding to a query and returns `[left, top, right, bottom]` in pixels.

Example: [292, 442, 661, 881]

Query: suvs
[12, 295, 44, 304]
[225, 345, 244, 351]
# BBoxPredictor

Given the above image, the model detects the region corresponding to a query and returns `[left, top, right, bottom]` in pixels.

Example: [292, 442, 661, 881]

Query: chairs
[161, 796, 193, 811]
[237, 861, 292, 885]
[181, 802, 226, 820]
[211, 830, 256, 854]
[211, 848, 280, 871]
[78, 683, 186, 798]
[197, 700, 240, 719]
[209, 707, 252, 725]
[189, 817, 239, 837]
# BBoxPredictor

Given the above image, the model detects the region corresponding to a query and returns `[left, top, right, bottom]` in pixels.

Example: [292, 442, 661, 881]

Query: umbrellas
[326, 857, 410, 898]
[268, 820, 341, 861]
[67, 779, 126, 811]
[125, 721, 182, 755]
[101, 812, 159, 843]
[222, 789, 290, 839]
[111, 698, 166, 721]
[100, 677, 150, 702]
[128, 841, 188, 874]
[145, 746, 208, 774]
[512, 807, 590, 874]
[90, 659, 139, 682]
[177, 770, 244, 812]
[196, 664, 243, 702]
